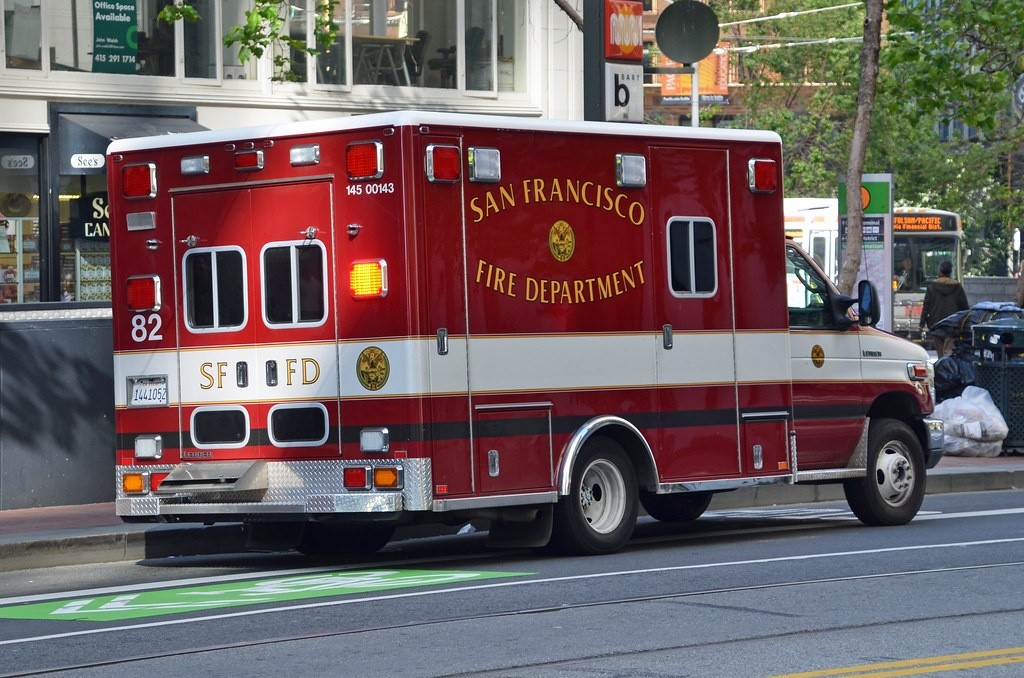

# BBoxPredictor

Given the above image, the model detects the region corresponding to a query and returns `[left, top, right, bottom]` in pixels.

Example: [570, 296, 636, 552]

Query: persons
[899, 259, 926, 290]
[918, 261, 969, 359]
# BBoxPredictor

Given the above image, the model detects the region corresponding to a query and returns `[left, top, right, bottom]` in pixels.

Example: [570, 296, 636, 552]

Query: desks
[289, 33, 420, 86]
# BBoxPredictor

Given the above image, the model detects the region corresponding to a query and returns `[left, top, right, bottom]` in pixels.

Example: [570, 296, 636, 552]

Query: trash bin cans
[971, 317, 1024, 455]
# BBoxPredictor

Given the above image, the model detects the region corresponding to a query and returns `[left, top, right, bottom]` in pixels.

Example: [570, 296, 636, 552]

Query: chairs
[427, 26, 484, 89]
[375, 30, 429, 86]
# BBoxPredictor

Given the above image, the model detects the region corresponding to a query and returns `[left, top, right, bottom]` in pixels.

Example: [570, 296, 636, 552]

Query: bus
[783, 198, 964, 340]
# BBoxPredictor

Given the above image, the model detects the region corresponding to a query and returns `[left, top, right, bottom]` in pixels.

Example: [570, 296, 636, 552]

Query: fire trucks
[113, 115, 946, 564]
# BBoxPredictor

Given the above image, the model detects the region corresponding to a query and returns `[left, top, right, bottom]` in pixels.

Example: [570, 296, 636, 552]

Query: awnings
[59, 111, 211, 176]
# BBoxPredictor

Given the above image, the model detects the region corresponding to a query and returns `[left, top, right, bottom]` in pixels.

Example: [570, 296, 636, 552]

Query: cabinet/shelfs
[0, 217, 111, 302]
